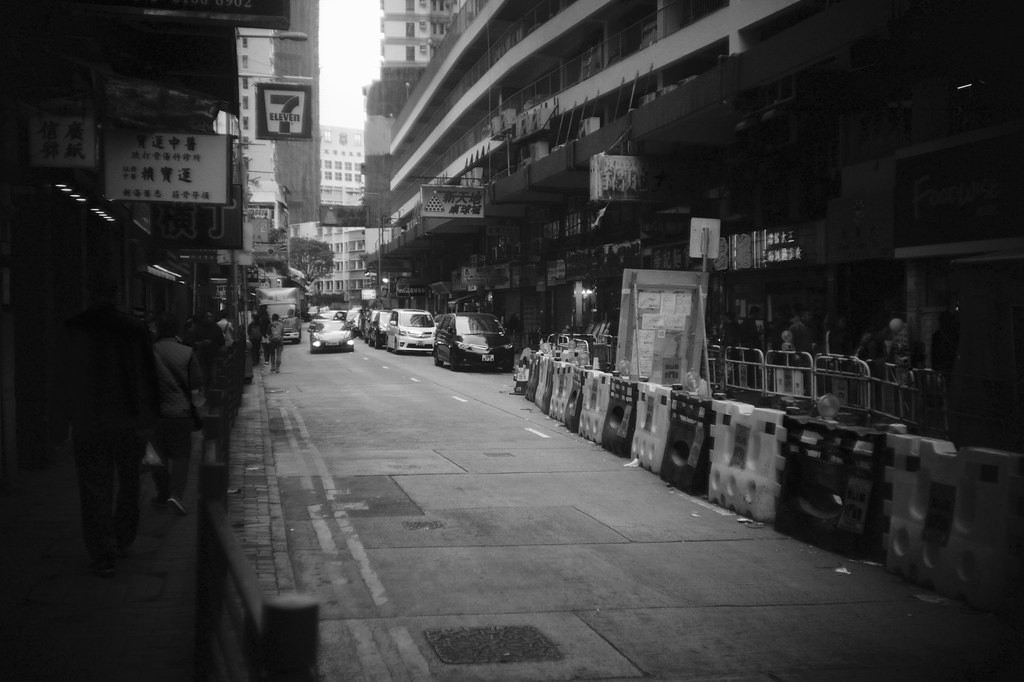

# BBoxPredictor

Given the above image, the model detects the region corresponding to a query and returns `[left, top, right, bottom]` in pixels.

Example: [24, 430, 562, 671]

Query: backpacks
[270, 323, 282, 342]
[249, 322, 261, 341]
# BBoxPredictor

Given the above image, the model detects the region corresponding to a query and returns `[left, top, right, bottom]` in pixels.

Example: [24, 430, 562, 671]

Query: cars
[433, 312, 514, 373]
[303, 293, 444, 354]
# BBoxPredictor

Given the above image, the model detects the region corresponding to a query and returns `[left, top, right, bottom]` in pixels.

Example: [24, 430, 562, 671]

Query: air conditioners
[419, 21, 427, 29]
[576, 117, 601, 139]
[418, 0, 426, 5]
[491, 108, 550, 169]
[420, 45, 427, 52]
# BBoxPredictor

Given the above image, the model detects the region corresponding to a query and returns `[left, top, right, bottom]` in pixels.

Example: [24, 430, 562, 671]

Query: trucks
[255, 289, 301, 343]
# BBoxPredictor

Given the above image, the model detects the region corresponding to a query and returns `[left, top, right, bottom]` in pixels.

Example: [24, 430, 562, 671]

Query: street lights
[346, 191, 382, 311]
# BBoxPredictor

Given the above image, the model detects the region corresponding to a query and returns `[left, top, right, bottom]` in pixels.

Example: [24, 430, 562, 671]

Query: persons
[63, 269, 284, 578]
[721, 309, 890, 380]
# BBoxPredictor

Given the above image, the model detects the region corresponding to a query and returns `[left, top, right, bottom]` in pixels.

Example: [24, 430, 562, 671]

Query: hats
[152, 311, 178, 337]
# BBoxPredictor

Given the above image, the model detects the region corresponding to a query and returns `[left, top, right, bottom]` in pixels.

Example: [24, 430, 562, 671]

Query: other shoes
[87, 563, 116, 576]
[165, 496, 187, 515]
[275, 370, 280, 373]
[110, 543, 128, 557]
[264, 361, 269, 365]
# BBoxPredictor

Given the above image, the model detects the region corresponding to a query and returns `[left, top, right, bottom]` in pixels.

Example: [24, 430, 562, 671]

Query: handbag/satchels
[191, 396, 208, 430]
[140, 440, 164, 474]
